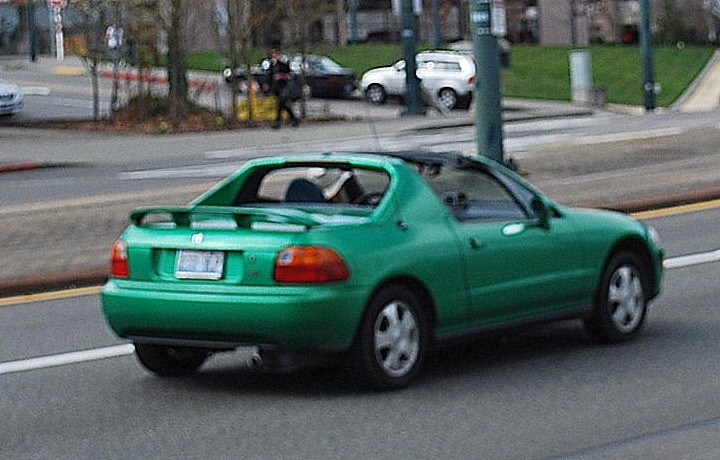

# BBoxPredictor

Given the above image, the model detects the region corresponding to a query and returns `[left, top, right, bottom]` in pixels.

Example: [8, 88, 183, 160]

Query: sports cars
[98, 147, 670, 394]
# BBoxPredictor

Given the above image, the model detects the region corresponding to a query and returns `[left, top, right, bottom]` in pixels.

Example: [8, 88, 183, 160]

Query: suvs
[361, 49, 483, 112]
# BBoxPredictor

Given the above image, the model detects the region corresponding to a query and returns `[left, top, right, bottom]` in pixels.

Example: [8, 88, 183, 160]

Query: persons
[126, 35, 137, 67]
[265, 44, 301, 129]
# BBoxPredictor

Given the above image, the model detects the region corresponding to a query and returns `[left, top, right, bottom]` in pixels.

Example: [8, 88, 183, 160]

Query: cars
[222, 52, 357, 98]
[0, 77, 24, 116]
